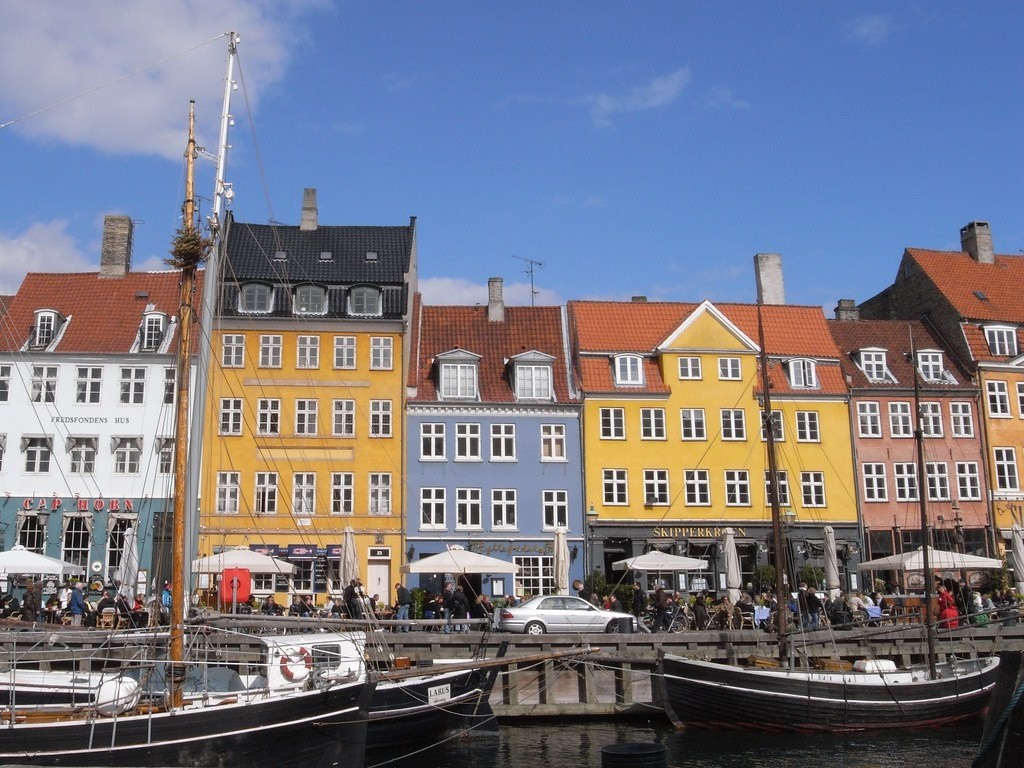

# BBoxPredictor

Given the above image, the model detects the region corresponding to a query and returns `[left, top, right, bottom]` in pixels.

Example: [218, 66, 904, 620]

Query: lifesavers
[281, 647, 312, 682]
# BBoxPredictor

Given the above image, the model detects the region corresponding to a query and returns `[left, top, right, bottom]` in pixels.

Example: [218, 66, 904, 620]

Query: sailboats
[0, 27, 1003, 768]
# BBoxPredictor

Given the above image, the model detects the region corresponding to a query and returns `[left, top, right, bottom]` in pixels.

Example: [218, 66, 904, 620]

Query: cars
[497, 595, 639, 635]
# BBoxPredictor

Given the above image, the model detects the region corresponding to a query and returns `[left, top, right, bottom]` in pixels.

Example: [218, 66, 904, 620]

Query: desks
[41, 609, 59, 624]
[374, 610, 393, 619]
[865, 606, 883, 627]
[705, 609, 720, 631]
[754, 608, 770, 627]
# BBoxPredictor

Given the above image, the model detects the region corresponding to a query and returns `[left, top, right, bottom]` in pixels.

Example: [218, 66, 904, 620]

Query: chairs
[287, 606, 347, 634]
[683, 606, 835, 632]
[424, 607, 446, 632]
[61, 607, 158, 632]
[7, 611, 23, 632]
[851, 605, 921, 628]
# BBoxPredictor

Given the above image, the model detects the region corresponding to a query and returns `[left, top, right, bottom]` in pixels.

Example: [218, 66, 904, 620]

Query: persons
[578, 569, 1016, 634]
[230, 578, 527, 634]
[0, 571, 172, 628]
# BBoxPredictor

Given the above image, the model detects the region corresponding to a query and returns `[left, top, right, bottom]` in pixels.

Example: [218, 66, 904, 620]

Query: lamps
[573, 544, 578, 556]
[410, 544, 416, 557]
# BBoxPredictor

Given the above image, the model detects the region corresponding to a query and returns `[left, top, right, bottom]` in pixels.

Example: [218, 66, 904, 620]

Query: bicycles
[641, 606, 688, 634]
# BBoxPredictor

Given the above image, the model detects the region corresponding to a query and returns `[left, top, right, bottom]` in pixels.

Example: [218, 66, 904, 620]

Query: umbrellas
[400, 545, 520, 590]
[1012, 524, 1024, 600]
[0, 543, 83, 588]
[823, 525, 841, 602]
[612, 551, 708, 590]
[192, 546, 297, 574]
[856, 546, 1004, 571]
[722, 527, 743, 604]
[337, 526, 361, 587]
[115, 528, 139, 608]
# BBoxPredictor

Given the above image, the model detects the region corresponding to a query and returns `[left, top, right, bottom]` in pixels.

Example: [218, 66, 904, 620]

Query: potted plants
[985, 550, 1022, 627]
